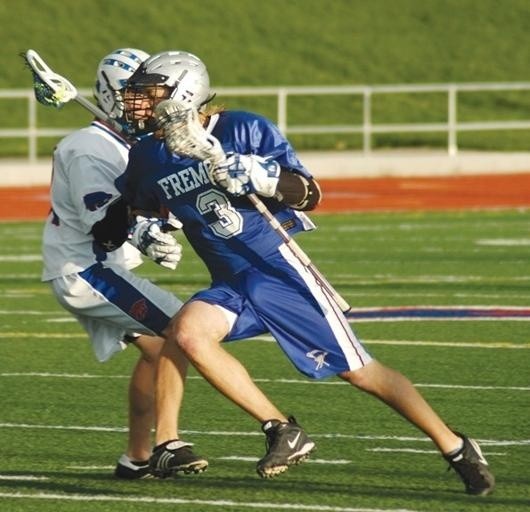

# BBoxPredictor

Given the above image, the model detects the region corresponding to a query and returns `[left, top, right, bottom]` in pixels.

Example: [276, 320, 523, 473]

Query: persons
[38, 45, 211, 483]
[113, 49, 497, 498]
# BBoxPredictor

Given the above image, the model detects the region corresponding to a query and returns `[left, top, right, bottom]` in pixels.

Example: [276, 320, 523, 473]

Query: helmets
[93, 46, 216, 140]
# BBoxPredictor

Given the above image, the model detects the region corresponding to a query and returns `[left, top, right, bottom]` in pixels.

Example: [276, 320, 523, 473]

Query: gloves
[128, 213, 183, 270]
[214, 150, 281, 199]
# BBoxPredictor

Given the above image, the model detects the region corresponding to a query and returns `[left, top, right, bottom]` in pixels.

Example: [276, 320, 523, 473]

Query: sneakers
[442, 429, 495, 495]
[256, 415, 315, 478]
[114, 439, 208, 480]
